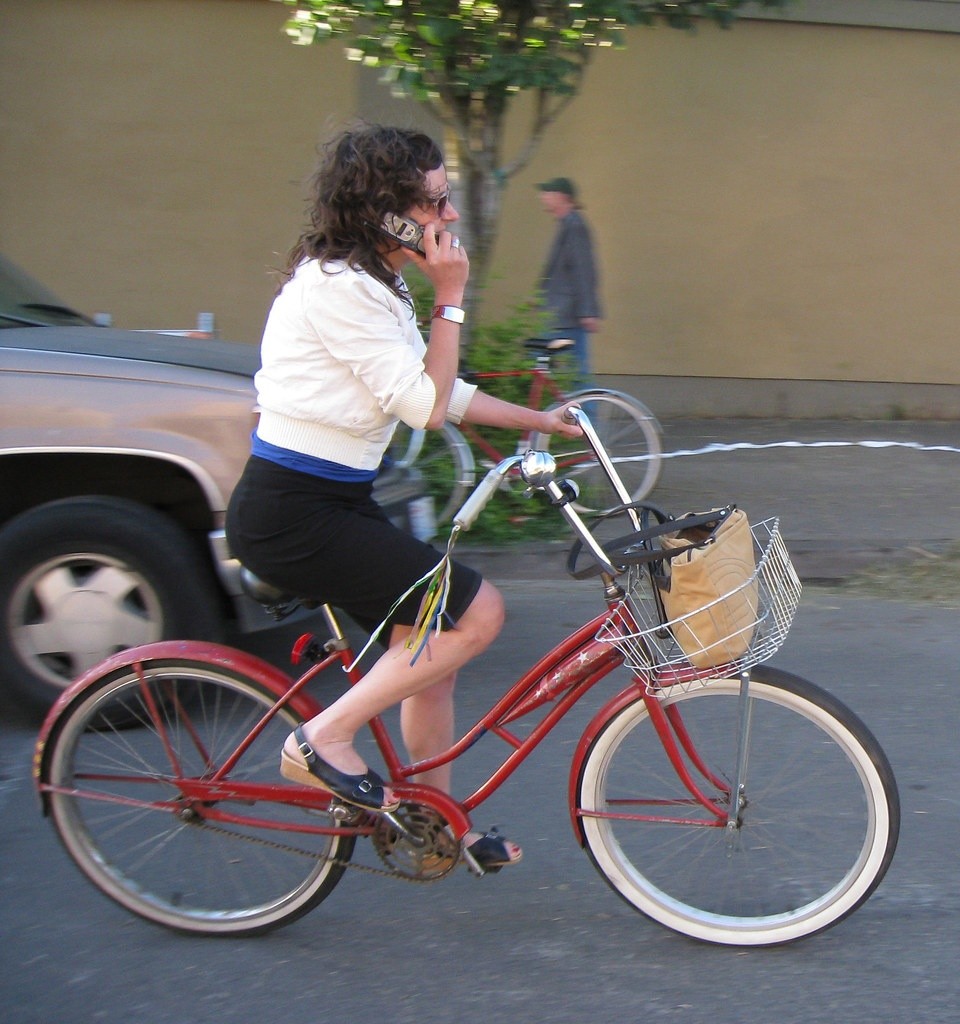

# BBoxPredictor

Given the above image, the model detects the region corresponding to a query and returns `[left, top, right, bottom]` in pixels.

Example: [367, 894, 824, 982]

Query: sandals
[280, 721, 401, 812]
[420, 827, 522, 874]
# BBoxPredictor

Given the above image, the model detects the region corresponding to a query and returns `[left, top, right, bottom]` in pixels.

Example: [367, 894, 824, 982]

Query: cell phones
[364, 209, 439, 259]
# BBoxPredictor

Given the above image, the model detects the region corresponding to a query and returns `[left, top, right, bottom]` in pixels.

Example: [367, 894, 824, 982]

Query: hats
[535, 177, 578, 196]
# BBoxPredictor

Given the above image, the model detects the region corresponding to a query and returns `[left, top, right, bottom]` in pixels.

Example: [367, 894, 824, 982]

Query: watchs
[429, 305, 466, 325]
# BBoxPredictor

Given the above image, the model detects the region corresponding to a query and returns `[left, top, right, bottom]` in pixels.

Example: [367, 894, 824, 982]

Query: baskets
[595, 516, 804, 698]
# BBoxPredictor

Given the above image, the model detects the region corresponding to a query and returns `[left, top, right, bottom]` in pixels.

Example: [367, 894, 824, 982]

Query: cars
[0, 245, 418, 736]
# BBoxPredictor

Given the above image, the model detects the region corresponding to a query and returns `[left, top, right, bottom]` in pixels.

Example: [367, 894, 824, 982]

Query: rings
[453, 238, 461, 248]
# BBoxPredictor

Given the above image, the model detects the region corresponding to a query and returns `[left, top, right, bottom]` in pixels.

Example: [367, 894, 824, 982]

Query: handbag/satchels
[567, 500, 758, 669]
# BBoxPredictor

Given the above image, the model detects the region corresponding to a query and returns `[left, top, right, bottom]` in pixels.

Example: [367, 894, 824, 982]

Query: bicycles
[383, 316, 667, 535]
[28, 405, 904, 948]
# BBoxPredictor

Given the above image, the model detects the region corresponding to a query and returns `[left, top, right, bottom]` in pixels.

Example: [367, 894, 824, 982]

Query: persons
[222, 125, 588, 868]
[528, 175, 604, 436]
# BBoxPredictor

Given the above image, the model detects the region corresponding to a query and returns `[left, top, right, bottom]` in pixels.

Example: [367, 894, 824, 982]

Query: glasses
[409, 185, 451, 218]
